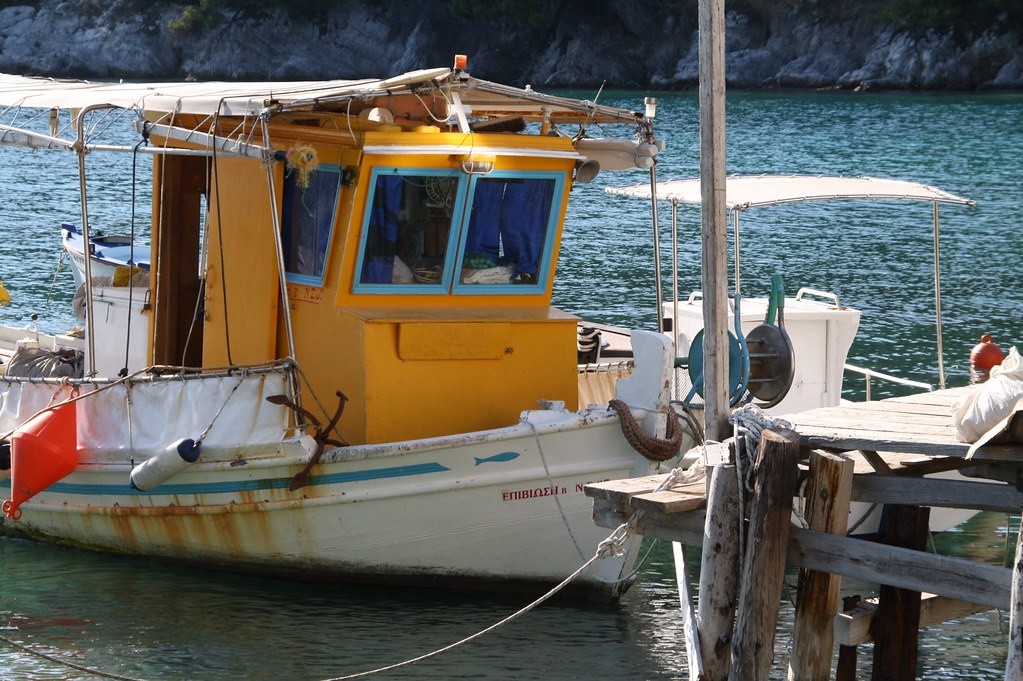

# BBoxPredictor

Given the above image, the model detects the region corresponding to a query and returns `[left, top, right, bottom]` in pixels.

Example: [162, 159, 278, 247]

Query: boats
[600, 175, 1023, 534]
[55, 222, 634, 363]
[0, 47, 682, 601]
